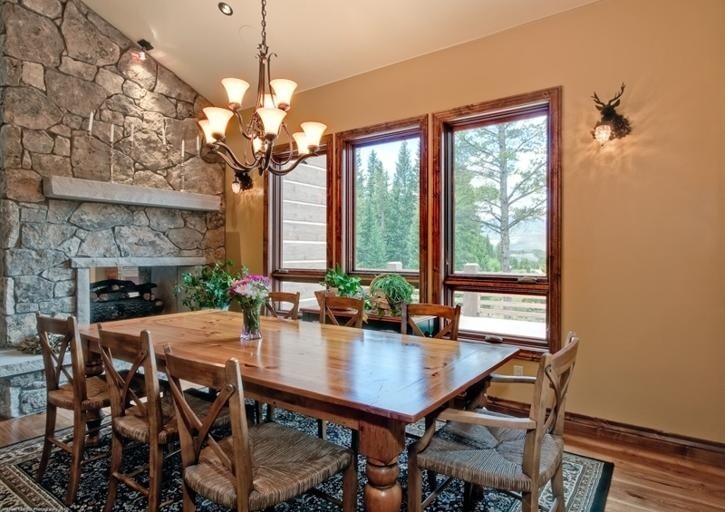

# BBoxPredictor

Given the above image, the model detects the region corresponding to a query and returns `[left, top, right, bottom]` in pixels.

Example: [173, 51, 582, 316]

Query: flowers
[228, 276, 273, 331]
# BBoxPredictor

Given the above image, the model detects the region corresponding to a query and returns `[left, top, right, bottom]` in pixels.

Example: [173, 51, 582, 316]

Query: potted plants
[173, 259, 248, 311]
[321, 264, 363, 310]
[368, 274, 415, 316]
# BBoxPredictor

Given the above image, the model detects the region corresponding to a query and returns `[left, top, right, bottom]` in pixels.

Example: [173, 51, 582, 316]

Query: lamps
[231, 163, 253, 194]
[196, 1, 328, 176]
[136, 39, 154, 59]
[588, 82, 633, 146]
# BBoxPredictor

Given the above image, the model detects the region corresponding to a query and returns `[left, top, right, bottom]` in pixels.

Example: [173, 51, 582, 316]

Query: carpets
[0, 386, 614, 512]
